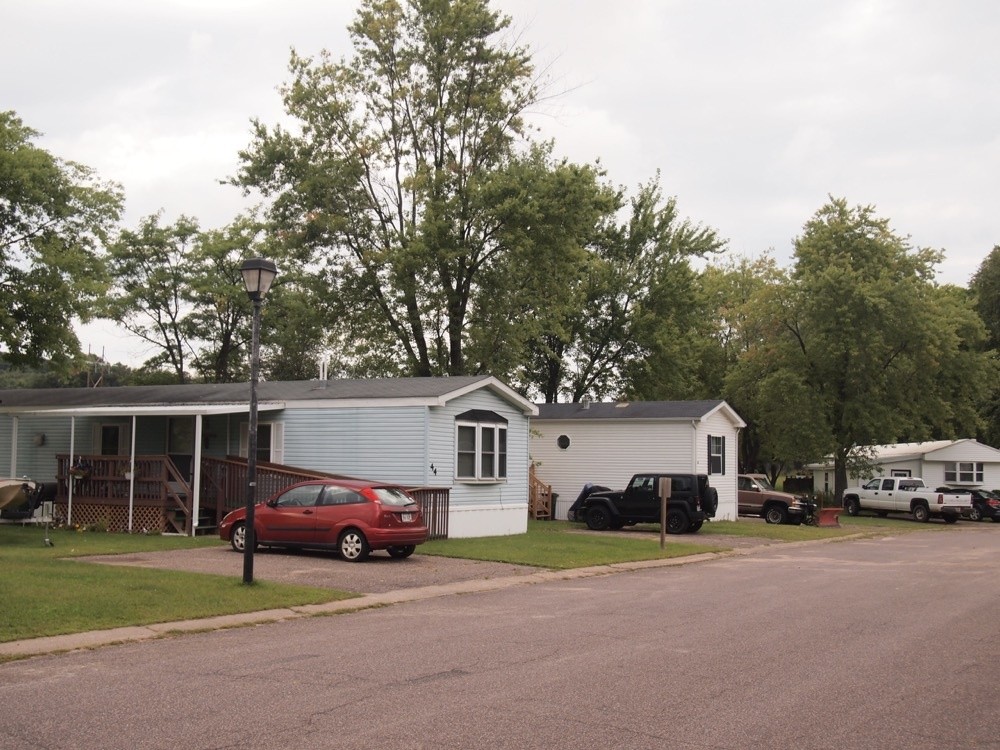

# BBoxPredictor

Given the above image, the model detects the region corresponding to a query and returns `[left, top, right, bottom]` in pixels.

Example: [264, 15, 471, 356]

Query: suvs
[583, 472, 719, 535]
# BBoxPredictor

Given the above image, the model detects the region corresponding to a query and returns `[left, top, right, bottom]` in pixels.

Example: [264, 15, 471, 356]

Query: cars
[737, 472, 809, 525]
[219, 479, 430, 563]
[950, 487, 1000, 524]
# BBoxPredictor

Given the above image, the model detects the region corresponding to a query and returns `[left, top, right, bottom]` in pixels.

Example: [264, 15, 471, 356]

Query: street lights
[238, 258, 279, 584]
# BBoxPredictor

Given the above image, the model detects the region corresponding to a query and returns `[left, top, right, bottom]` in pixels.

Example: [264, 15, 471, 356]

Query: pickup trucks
[842, 476, 974, 523]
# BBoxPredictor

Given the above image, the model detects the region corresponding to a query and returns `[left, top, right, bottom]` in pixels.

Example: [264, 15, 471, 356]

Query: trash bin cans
[541, 493, 560, 520]
